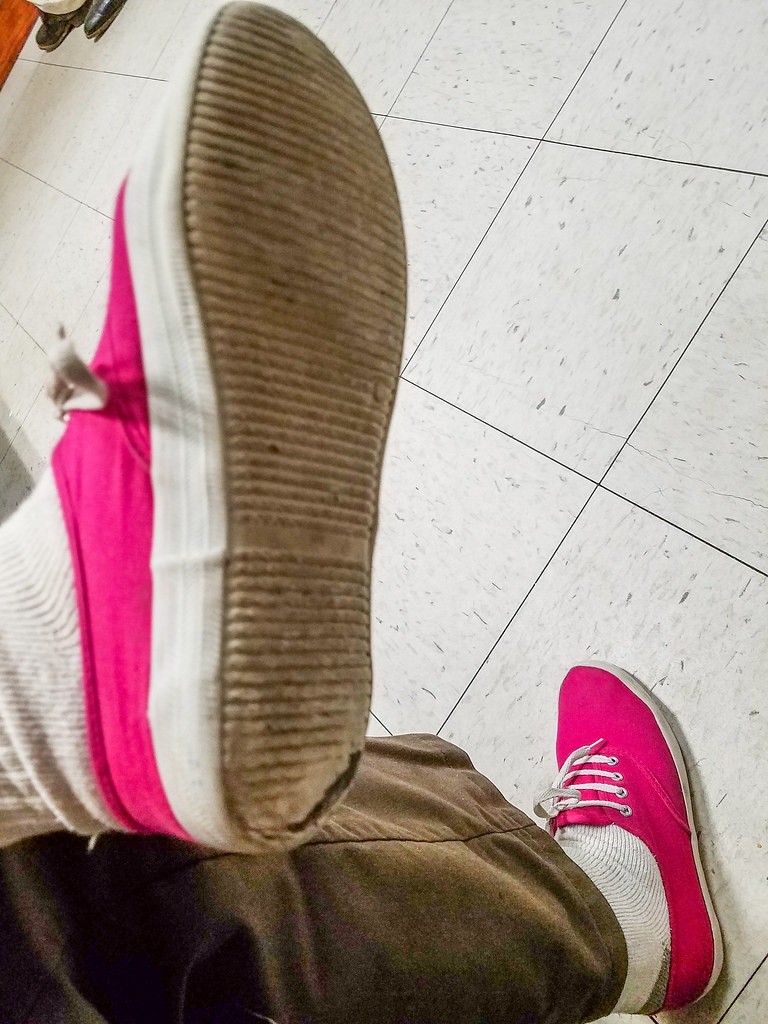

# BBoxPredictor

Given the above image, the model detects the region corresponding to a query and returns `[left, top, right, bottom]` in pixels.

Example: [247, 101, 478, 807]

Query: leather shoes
[36, 0, 92, 50]
[84, 0, 126, 39]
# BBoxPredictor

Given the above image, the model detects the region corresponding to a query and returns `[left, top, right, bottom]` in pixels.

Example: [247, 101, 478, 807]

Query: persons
[0, 0, 725, 1024]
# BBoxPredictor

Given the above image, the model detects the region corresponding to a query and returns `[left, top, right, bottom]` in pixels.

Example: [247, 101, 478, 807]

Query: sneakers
[48, 0, 408, 855]
[532, 658, 723, 1016]
